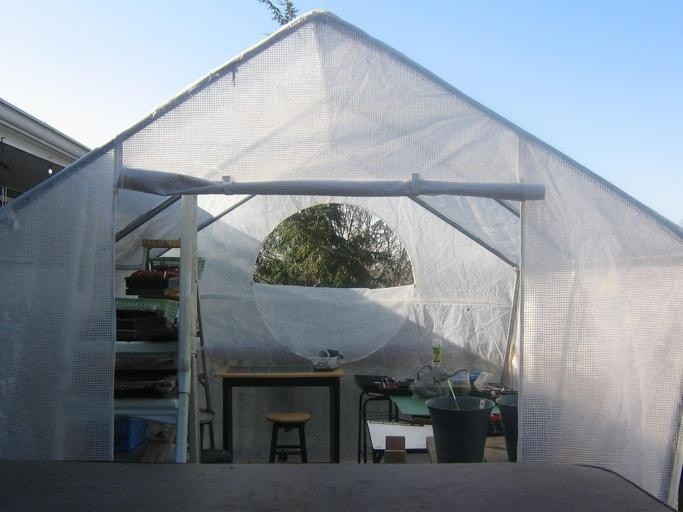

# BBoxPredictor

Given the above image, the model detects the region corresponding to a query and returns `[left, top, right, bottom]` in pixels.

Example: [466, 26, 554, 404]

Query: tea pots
[412, 361, 468, 396]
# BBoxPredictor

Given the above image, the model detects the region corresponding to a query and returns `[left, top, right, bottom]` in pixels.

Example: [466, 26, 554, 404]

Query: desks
[212, 360, 345, 462]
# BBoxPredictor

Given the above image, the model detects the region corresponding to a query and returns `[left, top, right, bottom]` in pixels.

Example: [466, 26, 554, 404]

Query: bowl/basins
[354, 375, 415, 396]
[310, 356, 341, 371]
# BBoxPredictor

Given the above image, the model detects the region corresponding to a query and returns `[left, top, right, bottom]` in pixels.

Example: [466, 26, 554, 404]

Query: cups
[496, 395, 558, 462]
[425, 392, 496, 462]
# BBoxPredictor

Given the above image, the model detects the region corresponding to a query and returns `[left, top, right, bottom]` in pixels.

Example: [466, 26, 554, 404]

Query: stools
[263, 412, 311, 463]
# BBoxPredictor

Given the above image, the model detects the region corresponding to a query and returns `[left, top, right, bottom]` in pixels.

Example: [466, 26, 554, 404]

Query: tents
[0, 9, 682, 512]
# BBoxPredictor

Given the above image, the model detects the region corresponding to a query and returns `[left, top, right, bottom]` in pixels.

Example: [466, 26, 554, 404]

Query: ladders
[140, 239, 216, 450]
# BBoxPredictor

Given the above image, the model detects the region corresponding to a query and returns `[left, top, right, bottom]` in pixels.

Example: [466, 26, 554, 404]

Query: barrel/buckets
[426, 395, 495, 463]
[495, 394, 518, 463]
